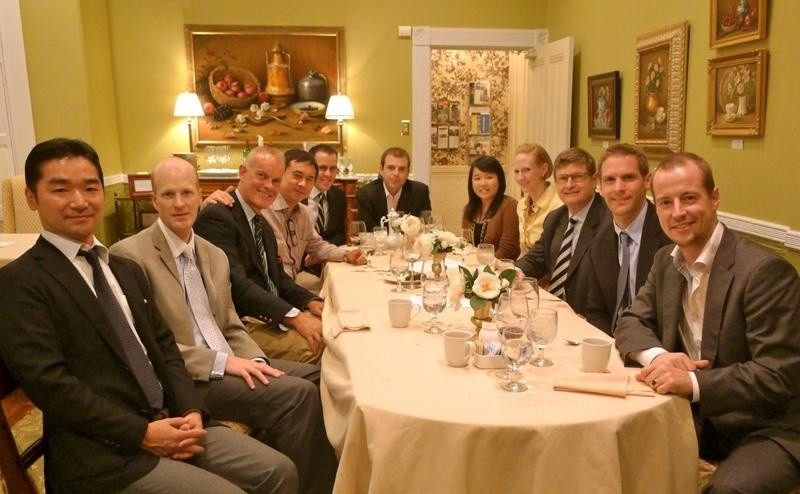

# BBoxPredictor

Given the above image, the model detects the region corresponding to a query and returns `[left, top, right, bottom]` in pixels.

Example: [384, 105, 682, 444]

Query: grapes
[213, 102, 232, 121]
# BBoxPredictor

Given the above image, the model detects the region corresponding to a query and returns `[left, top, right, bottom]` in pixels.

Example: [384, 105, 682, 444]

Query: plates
[291, 101, 326, 117]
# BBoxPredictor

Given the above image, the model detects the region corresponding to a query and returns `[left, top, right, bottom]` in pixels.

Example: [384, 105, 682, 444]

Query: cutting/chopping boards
[244, 106, 287, 125]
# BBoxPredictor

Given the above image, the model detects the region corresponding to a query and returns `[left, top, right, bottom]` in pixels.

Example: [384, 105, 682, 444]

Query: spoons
[566, 339, 581, 346]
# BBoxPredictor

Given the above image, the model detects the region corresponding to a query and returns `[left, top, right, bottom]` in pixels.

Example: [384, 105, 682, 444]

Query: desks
[198, 175, 358, 246]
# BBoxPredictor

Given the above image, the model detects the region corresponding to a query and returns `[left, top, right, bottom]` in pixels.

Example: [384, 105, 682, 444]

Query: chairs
[0, 387, 43, 494]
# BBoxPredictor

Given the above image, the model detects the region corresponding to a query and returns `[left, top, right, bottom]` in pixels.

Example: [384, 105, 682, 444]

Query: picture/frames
[184, 23, 344, 152]
[587, 71, 619, 140]
[633, 20, 689, 162]
[708, 0, 767, 49]
[706, 48, 768, 137]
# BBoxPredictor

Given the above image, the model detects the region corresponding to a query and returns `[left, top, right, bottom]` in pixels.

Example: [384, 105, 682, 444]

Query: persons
[355, 147, 432, 235]
[191, 145, 326, 366]
[614, 150, 799, 493]
[511, 148, 614, 319]
[298, 143, 348, 278]
[0, 139, 299, 494]
[460, 156, 521, 263]
[512, 141, 565, 266]
[109, 155, 338, 494]
[199, 150, 368, 296]
[585, 142, 675, 340]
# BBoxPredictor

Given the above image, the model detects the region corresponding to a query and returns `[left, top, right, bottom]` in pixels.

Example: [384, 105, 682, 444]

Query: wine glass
[723, 103, 737, 122]
[654, 107, 666, 124]
[346, 158, 354, 178]
[350, 209, 558, 393]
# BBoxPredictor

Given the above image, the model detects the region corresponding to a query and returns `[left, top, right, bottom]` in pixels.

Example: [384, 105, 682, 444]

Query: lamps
[173, 92, 205, 152]
[326, 95, 355, 156]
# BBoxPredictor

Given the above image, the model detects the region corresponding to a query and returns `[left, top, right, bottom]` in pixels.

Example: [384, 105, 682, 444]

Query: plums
[723, 12, 740, 26]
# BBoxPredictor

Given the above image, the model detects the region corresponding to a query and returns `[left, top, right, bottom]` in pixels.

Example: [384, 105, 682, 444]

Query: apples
[258, 91, 269, 104]
[744, 9, 757, 27]
[204, 102, 214, 114]
[216, 72, 255, 98]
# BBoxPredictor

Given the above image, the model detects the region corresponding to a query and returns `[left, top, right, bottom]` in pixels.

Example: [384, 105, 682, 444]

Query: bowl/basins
[721, 19, 738, 33]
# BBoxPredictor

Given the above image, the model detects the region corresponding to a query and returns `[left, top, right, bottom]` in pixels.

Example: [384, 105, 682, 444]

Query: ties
[182, 249, 236, 359]
[548, 217, 577, 301]
[76, 247, 166, 409]
[315, 193, 326, 235]
[252, 213, 280, 298]
[611, 232, 631, 338]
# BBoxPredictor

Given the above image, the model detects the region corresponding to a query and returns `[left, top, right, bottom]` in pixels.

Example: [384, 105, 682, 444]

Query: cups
[337, 157, 346, 178]
[206, 145, 233, 169]
[581, 338, 612, 373]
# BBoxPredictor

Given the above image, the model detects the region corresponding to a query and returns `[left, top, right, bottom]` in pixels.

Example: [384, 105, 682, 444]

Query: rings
[652, 379, 657, 389]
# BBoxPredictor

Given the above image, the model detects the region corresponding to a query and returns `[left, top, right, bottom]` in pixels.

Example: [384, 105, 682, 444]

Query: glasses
[555, 173, 593, 186]
[288, 218, 299, 247]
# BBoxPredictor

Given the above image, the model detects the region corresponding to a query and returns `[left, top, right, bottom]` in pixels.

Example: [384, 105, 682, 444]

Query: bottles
[470, 111, 477, 135]
[481, 114, 490, 132]
[297, 69, 329, 101]
[243, 138, 251, 164]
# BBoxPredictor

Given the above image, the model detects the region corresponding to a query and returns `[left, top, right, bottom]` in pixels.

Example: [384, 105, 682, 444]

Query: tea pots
[262, 41, 296, 108]
